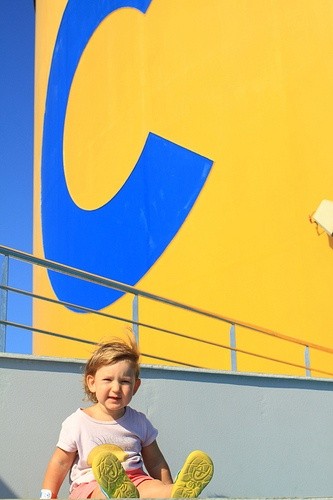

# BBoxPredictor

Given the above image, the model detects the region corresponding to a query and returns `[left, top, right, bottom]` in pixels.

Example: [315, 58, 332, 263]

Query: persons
[37, 331, 216, 500]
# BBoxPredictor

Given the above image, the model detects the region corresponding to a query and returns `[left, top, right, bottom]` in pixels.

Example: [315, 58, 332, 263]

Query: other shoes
[91, 451, 141, 499]
[167, 449, 214, 500]
